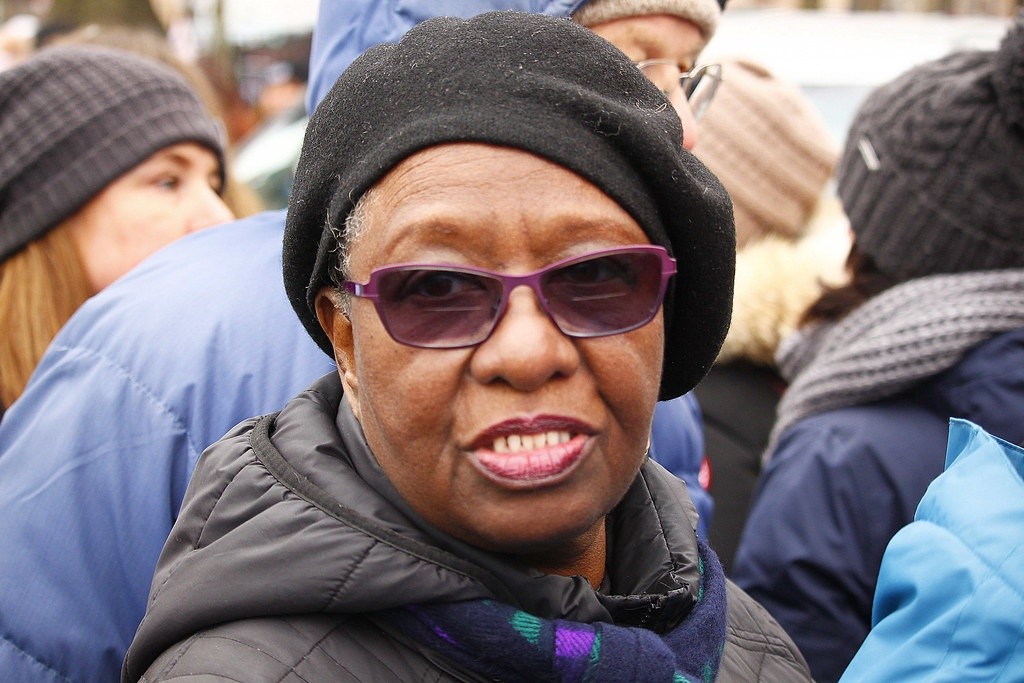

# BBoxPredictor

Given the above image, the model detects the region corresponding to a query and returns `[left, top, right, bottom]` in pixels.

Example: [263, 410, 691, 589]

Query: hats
[282, 12, 735, 402]
[0, 47, 226, 266]
[838, 25, 1023, 279]
[687, 58, 837, 240]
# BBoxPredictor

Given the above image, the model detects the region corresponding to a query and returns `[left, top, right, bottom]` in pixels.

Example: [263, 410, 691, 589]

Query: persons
[680, 59, 859, 572]
[119, 10, 818, 682]
[0, 0, 718, 682]
[0, 45, 242, 416]
[726, 23, 1024, 682]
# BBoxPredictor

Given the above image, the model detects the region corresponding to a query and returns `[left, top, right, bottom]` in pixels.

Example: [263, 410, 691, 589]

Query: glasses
[341, 244, 676, 349]
